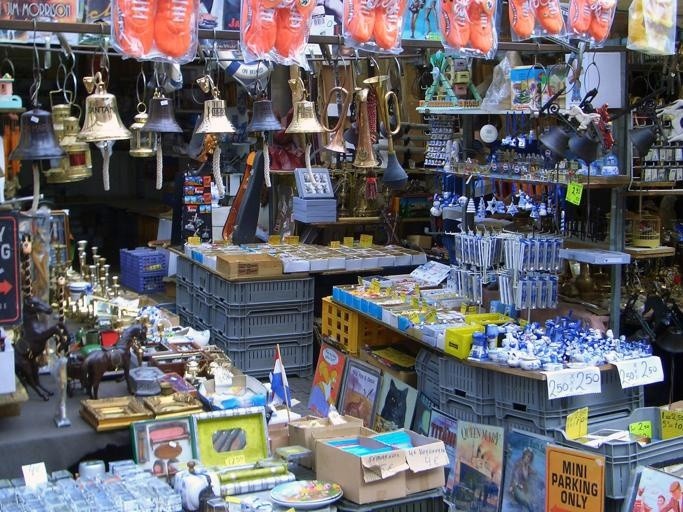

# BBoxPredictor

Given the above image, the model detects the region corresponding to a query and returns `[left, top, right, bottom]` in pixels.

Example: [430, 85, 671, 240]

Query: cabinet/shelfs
[0, 0, 683, 512]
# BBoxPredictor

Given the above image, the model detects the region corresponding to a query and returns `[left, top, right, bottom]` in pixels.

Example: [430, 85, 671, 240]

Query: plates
[197, 374, 268, 412]
[267, 479, 344, 510]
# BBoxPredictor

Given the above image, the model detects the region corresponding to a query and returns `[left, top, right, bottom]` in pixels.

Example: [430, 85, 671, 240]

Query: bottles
[262, 381, 273, 404]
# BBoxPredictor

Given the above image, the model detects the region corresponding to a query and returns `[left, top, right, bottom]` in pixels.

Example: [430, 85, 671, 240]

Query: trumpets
[321, 62, 349, 155]
[362, 58, 401, 136]
[362, 74, 410, 188]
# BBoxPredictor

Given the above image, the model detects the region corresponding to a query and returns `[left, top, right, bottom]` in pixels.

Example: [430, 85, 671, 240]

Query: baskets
[119, 246, 166, 294]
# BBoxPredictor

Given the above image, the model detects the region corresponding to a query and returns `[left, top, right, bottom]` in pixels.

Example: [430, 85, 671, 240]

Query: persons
[650, 495, 665, 512]
[661, 481, 683, 512]
[416, 408, 430, 437]
[507, 446, 540, 512]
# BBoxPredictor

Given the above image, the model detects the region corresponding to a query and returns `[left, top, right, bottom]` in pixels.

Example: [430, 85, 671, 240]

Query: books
[370, 372, 417, 434]
[304, 343, 346, 419]
[426, 406, 461, 506]
[499, 428, 555, 511]
[412, 391, 442, 439]
[620, 464, 682, 512]
[335, 356, 381, 431]
[544, 443, 605, 512]
[453, 420, 504, 511]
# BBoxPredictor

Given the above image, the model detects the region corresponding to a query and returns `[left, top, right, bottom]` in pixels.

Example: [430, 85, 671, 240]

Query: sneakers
[240, 0, 278, 54]
[570, 0, 592, 33]
[648, 1, 673, 54]
[536, 0, 564, 33]
[343, 0, 375, 42]
[111, 0, 156, 57]
[467, 0, 495, 54]
[509, 0, 535, 38]
[274, 0, 316, 58]
[374, 0, 406, 49]
[588, 0, 617, 41]
[440, 0, 469, 49]
[155, 0, 193, 58]
[628, 1, 649, 51]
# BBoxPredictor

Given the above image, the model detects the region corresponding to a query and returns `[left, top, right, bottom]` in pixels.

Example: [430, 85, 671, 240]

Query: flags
[268, 346, 291, 408]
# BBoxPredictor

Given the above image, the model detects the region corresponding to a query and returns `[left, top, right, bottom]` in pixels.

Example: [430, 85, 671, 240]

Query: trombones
[352, 60, 379, 169]
